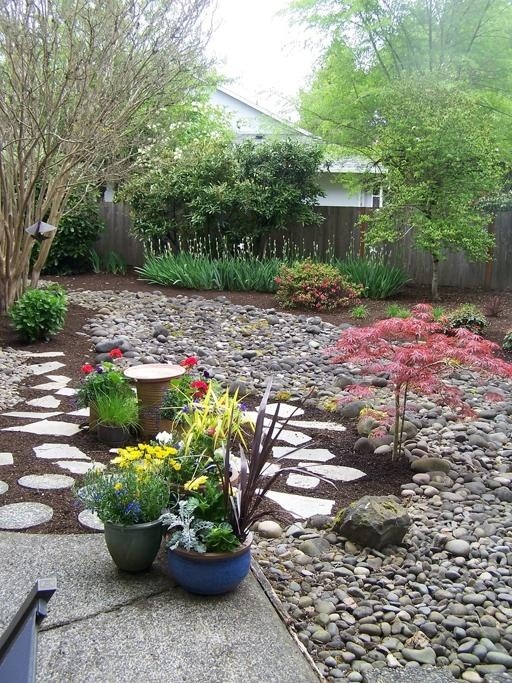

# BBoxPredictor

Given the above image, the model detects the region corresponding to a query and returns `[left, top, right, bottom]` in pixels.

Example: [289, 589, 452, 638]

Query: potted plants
[165, 376, 339, 596]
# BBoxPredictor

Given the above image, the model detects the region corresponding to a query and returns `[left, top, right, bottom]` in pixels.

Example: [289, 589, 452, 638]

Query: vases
[103, 514, 166, 572]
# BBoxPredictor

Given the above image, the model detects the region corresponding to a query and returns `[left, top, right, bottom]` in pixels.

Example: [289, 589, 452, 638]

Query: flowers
[71, 349, 256, 525]
